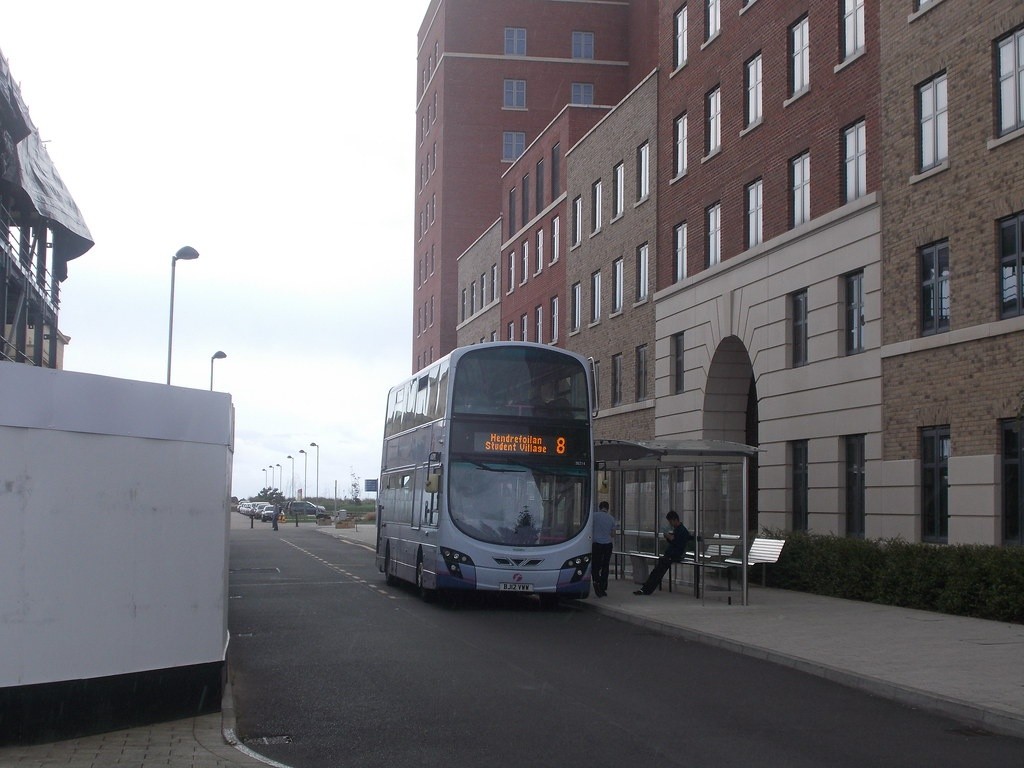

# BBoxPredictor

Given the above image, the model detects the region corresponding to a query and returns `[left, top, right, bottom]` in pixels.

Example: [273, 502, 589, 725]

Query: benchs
[611, 532, 786, 606]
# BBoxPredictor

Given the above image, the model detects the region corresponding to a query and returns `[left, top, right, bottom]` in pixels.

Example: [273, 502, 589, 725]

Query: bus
[376, 342, 596, 607]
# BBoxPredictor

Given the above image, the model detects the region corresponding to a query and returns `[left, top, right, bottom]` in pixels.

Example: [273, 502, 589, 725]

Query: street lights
[310, 443, 319, 515]
[276, 464, 282, 494]
[287, 456, 294, 498]
[262, 468, 270, 490]
[269, 466, 274, 489]
[299, 450, 307, 500]
[209, 352, 227, 391]
[166, 246, 199, 385]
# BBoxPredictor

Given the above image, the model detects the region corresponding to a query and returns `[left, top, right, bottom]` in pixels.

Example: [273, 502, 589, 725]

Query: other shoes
[595, 584, 654, 598]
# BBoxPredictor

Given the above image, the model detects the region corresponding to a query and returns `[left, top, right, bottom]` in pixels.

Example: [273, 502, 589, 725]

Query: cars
[254, 504, 268, 519]
[261, 506, 274, 522]
[237, 501, 270, 517]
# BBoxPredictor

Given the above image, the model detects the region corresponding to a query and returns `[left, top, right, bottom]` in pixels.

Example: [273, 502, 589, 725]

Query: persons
[633, 510, 689, 596]
[272, 502, 280, 531]
[593, 501, 615, 597]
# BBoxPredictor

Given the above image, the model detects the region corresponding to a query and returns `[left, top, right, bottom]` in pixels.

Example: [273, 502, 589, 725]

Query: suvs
[288, 501, 326, 515]
